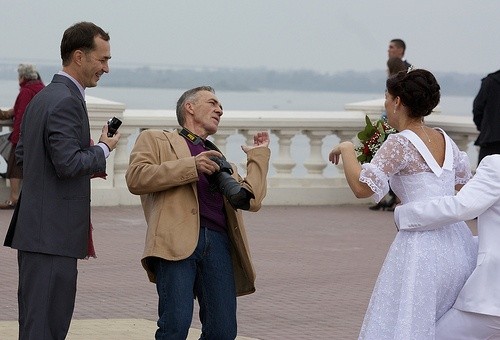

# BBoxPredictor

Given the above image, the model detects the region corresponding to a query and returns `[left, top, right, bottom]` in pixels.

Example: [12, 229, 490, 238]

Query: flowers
[354, 114, 398, 165]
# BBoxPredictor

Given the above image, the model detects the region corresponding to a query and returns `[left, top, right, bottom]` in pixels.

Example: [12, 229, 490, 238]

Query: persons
[393, 69, 500, 340]
[381, 38, 411, 209]
[126, 85, 271, 340]
[366, 55, 405, 212]
[327, 69, 478, 340]
[3, 21, 121, 340]
[0, 63, 45, 209]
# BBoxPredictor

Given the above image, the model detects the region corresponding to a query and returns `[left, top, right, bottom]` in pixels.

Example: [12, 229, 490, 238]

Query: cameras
[106, 117, 122, 138]
[203, 156, 256, 213]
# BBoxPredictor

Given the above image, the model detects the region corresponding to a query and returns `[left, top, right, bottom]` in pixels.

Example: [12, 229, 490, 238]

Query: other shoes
[0, 200, 19, 210]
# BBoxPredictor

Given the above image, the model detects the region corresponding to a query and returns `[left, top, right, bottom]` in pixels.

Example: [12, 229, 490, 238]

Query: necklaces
[420, 126, 431, 143]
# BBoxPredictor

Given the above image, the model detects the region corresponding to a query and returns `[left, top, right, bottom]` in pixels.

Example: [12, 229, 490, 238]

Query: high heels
[369, 200, 387, 212]
